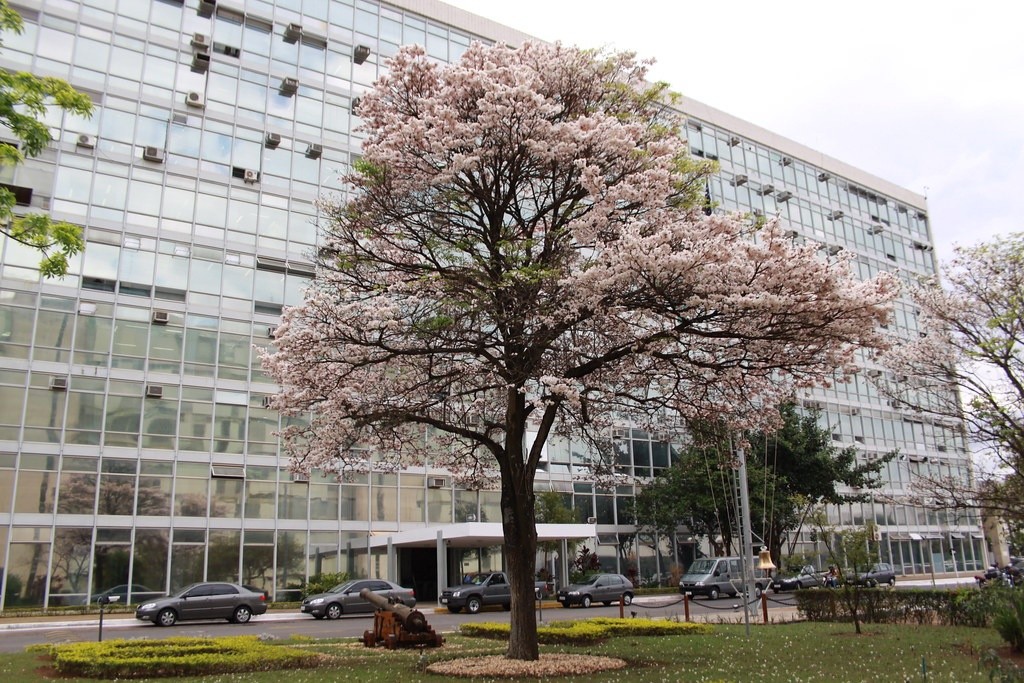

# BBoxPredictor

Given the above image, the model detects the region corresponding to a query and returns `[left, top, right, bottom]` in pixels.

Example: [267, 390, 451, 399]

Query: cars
[846, 562, 896, 587]
[300, 578, 417, 620]
[555, 573, 637, 608]
[81, 583, 153, 605]
[134, 581, 269, 628]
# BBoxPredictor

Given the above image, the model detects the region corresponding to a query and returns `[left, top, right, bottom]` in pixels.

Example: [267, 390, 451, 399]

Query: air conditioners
[308, 143, 323, 156]
[143, 145, 164, 162]
[874, 225, 884, 234]
[823, 172, 831, 179]
[428, 477, 446, 489]
[77, 134, 95, 148]
[872, 370, 882, 377]
[784, 157, 791, 164]
[588, 517, 596, 523]
[733, 137, 740, 143]
[818, 402, 825, 409]
[873, 524, 883, 540]
[354, 45, 370, 59]
[152, 311, 168, 322]
[287, 23, 302, 37]
[899, 376, 908, 381]
[764, 184, 774, 193]
[738, 175, 748, 181]
[354, 97, 361, 106]
[193, 33, 210, 47]
[466, 514, 476, 522]
[786, 230, 798, 238]
[52, 377, 68, 388]
[263, 396, 273, 406]
[834, 210, 845, 218]
[807, 402, 814, 408]
[267, 133, 281, 144]
[835, 246, 843, 251]
[294, 472, 309, 482]
[268, 326, 282, 337]
[146, 385, 163, 397]
[780, 190, 792, 200]
[894, 399, 903, 408]
[938, 443, 946, 450]
[283, 78, 299, 90]
[245, 169, 257, 181]
[852, 408, 860, 414]
[187, 91, 205, 106]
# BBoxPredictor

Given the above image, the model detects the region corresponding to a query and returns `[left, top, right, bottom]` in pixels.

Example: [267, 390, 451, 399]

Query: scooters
[974, 556, 1024, 590]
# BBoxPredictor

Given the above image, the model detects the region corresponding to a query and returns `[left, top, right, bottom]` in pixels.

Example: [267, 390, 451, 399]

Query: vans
[678, 554, 771, 601]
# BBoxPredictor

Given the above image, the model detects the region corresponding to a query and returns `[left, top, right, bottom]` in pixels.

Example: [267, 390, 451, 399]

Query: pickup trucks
[769, 564, 832, 594]
[439, 571, 550, 614]
